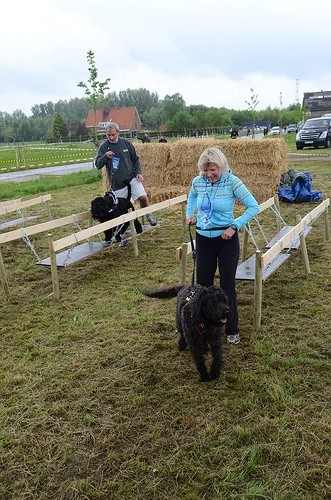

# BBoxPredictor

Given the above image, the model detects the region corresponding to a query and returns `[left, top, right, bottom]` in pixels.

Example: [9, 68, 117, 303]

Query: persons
[95, 122, 156, 226]
[186, 147, 260, 345]
[230, 127, 269, 139]
[135, 132, 150, 143]
[159, 135, 167, 143]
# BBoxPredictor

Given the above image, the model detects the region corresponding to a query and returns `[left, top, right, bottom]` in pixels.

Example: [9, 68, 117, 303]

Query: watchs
[231, 224, 238, 232]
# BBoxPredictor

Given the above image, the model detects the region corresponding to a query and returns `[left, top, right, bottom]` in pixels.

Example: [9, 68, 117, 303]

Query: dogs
[144, 283, 231, 382]
[91, 180, 143, 242]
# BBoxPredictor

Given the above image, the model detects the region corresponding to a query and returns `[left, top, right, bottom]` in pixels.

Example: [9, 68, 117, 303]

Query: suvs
[286, 123, 298, 133]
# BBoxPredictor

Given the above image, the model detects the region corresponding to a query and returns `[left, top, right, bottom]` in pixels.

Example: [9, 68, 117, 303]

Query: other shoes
[227, 334, 240, 345]
[146, 213, 157, 226]
[119, 239, 128, 248]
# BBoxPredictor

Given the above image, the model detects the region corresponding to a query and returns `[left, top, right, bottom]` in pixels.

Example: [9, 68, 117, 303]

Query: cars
[270, 127, 280, 135]
[295, 117, 331, 151]
